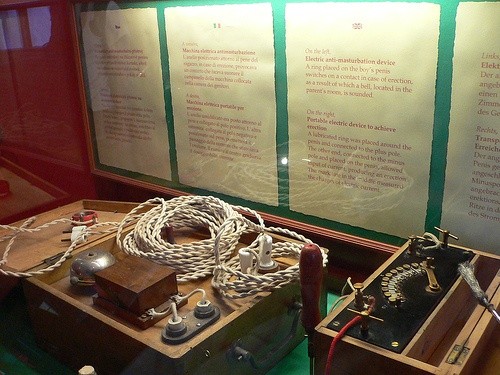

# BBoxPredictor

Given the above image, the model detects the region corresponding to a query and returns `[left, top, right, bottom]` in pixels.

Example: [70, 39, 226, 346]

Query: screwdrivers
[457, 263, 500, 323]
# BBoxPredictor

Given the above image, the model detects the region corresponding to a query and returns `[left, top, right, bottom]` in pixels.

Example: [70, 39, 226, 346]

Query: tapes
[70, 225, 87, 245]
